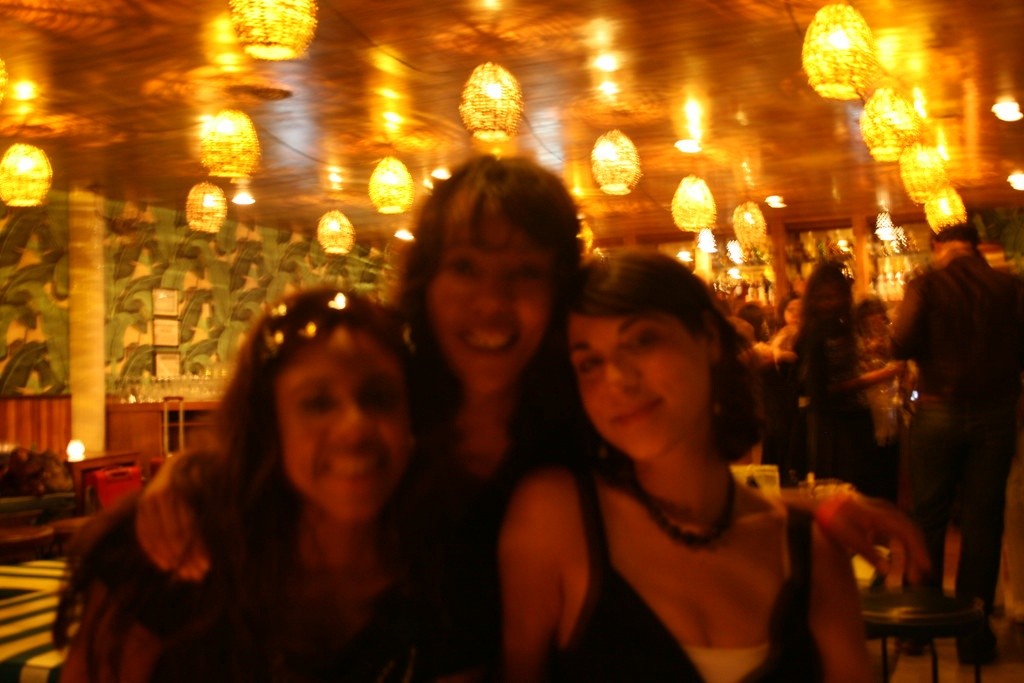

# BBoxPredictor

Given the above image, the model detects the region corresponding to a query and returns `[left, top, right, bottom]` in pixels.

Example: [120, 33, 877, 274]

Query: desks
[62, 449, 140, 512]
[0, 556, 70, 683]
[107, 401, 222, 459]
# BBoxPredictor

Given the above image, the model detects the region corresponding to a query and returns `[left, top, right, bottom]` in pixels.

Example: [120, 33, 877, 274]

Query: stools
[0, 526, 55, 564]
[859, 594, 986, 683]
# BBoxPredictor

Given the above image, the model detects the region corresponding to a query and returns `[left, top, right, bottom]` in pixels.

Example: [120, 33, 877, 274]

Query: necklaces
[636, 471, 736, 544]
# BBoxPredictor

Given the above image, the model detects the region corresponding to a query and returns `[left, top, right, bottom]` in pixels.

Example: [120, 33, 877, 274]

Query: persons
[711, 221, 1024, 666]
[497, 251, 886, 683]
[132, 152, 930, 683]
[57, 288, 489, 683]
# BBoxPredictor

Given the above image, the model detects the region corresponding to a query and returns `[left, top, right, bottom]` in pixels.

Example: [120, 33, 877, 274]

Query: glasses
[258, 290, 372, 365]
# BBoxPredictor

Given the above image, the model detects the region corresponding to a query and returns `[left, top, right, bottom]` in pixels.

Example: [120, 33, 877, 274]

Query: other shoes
[895, 622, 928, 654]
[957, 624, 997, 667]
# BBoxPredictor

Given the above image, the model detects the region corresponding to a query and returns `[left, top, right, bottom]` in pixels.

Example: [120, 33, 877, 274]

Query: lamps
[591, 127, 643, 195]
[802, 3, 879, 100]
[228, 0, 319, 62]
[316, 209, 356, 256]
[900, 141, 947, 204]
[0, 143, 53, 207]
[184, 181, 228, 234]
[367, 156, 416, 214]
[733, 201, 766, 249]
[200, 107, 261, 178]
[924, 186, 968, 240]
[858, 88, 922, 165]
[671, 174, 716, 234]
[457, 61, 523, 143]
